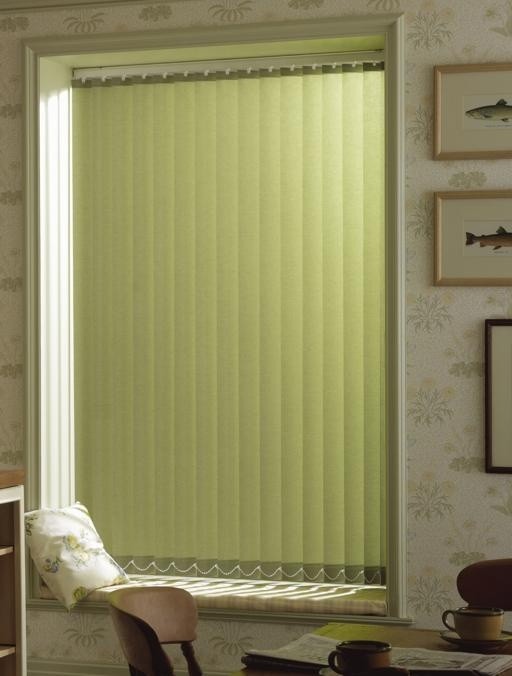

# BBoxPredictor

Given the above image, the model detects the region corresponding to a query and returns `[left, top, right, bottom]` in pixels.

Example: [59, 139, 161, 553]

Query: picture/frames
[484, 318, 511, 475]
[433, 63, 512, 161]
[434, 190, 511, 287]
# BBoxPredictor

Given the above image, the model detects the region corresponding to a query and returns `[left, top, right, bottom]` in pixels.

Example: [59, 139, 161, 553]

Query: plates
[439, 630, 512, 648]
[319, 666, 410, 676]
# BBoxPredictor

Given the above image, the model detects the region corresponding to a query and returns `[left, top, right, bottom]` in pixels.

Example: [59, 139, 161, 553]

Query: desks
[234, 619, 512, 676]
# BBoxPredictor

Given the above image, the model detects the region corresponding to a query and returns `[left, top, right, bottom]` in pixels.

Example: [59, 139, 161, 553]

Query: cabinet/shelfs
[0, 485, 27, 676]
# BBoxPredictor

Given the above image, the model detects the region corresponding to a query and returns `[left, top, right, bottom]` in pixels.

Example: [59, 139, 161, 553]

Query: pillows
[24, 501, 129, 611]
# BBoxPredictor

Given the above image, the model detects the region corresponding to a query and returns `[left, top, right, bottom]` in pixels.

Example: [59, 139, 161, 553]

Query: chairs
[108, 587, 205, 676]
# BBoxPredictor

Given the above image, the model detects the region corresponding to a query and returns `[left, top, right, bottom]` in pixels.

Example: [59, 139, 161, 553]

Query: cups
[442, 606, 505, 640]
[328, 640, 393, 675]
